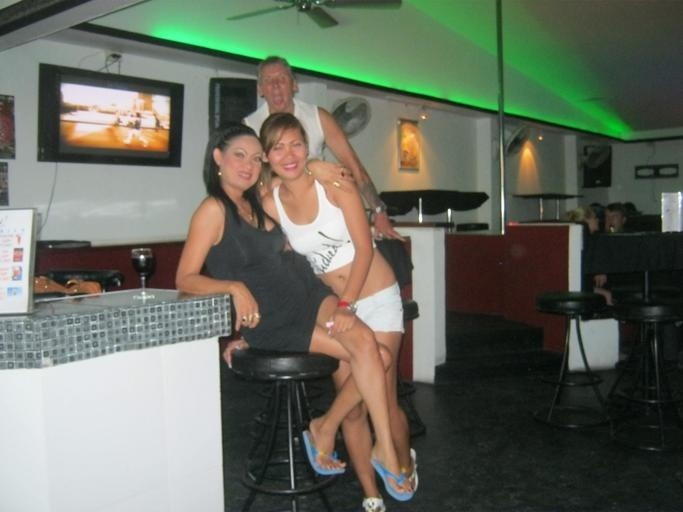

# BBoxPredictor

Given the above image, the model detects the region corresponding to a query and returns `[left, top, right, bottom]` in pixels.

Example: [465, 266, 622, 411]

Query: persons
[603, 202, 628, 233]
[255, 111, 421, 511]
[174, 122, 416, 503]
[564, 204, 616, 309]
[241, 54, 410, 246]
[113, 110, 165, 148]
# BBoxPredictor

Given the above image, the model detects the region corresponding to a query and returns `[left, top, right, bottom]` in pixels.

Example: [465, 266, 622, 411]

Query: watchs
[337, 300, 359, 313]
[369, 203, 386, 214]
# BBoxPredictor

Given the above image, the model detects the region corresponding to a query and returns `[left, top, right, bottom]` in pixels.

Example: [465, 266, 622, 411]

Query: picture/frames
[395, 116, 422, 172]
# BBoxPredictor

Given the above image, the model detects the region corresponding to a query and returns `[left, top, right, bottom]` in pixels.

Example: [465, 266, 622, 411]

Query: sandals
[303, 430, 345, 475]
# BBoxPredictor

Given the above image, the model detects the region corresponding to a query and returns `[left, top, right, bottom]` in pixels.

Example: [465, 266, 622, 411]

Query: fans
[325, 96, 372, 137]
[225, 0, 401, 28]
[498, 126, 529, 155]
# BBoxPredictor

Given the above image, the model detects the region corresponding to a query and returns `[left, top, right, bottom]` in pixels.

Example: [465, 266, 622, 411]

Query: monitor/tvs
[37, 62, 185, 167]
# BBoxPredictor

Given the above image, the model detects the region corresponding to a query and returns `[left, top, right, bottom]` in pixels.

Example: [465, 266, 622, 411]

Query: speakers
[208, 77, 258, 154]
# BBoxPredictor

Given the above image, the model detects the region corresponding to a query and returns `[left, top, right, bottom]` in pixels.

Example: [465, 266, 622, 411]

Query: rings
[253, 313, 261, 319]
[331, 180, 340, 188]
[247, 314, 253, 323]
[338, 168, 346, 178]
[241, 314, 246, 322]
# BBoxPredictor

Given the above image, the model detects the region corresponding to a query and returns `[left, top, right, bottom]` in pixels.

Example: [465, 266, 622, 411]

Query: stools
[228, 346, 341, 512]
[534, 291, 615, 431]
[606, 300, 682, 451]
[337, 301, 426, 445]
[606, 292, 682, 402]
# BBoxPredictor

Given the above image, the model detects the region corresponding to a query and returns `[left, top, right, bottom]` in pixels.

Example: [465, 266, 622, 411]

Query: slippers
[409, 448, 418, 491]
[371, 460, 415, 501]
[362, 495, 385, 511]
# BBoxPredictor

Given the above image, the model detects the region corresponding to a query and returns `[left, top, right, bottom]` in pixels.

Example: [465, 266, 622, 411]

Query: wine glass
[130, 248, 157, 302]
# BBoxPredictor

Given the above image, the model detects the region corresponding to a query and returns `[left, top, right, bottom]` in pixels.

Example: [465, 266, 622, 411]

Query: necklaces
[234, 199, 254, 222]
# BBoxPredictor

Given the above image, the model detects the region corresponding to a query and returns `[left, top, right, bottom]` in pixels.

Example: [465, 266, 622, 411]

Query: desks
[591, 232, 683, 418]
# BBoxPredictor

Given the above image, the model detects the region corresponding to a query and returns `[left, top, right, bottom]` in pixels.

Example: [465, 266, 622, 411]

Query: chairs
[34, 268, 107, 300]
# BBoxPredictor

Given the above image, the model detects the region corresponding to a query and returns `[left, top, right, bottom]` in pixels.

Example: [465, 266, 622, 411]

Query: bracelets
[303, 164, 313, 177]
[339, 294, 356, 303]
